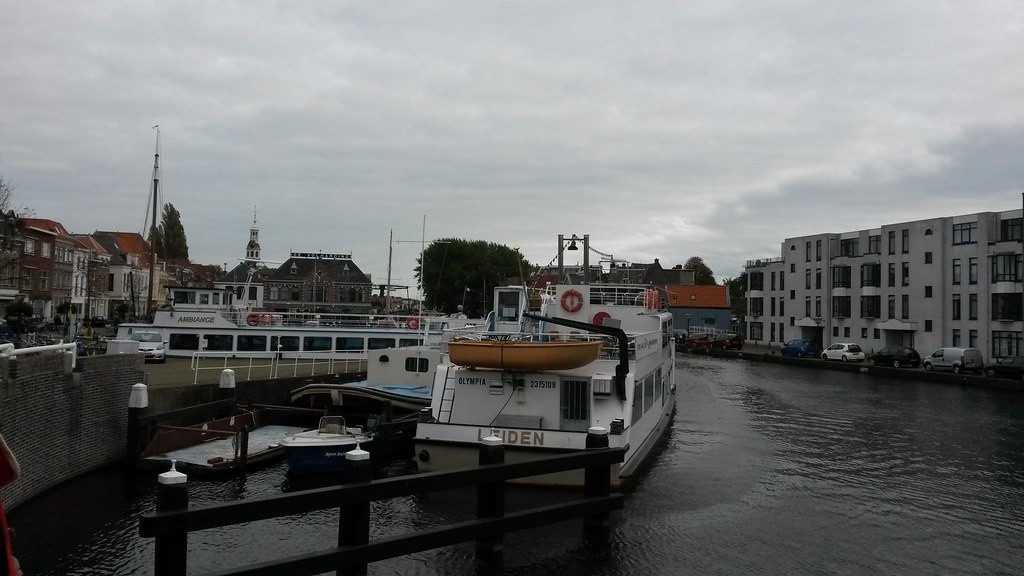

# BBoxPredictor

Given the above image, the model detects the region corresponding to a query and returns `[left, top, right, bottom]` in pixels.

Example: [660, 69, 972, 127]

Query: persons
[53, 314, 62, 334]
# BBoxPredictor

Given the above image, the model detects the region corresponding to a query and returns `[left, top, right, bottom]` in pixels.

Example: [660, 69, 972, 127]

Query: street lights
[685, 314, 692, 337]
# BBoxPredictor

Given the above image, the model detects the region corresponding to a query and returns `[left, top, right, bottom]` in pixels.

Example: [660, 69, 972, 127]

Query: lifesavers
[646, 288, 660, 310]
[246, 313, 271, 326]
[406, 317, 419, 329]
[561, 288, 584, 312]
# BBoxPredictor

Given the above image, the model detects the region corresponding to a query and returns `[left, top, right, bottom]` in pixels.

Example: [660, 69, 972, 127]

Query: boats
[85, 124, 559, 482]
[412, 281, 676, 495]
[448, 336, 604, 372]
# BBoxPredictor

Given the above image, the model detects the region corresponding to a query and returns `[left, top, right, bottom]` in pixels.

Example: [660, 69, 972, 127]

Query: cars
[128, 331, 170, 364]
[984, 355, 1024, 381]
[868, 346, 921, 368]
[781, 338, 822, 359]
[88, 315, 113, 329]
[75, 341, 86, 358]
[674, 332, 686, 345]
[820, 342, 866, 362]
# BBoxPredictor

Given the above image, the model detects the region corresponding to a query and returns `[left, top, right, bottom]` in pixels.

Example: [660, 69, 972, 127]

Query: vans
[922, 346, 983, 374]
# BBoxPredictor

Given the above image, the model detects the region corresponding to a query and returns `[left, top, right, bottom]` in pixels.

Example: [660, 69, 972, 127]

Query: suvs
[685, 333, 712, 348]
[708, 333, 745, 351]
